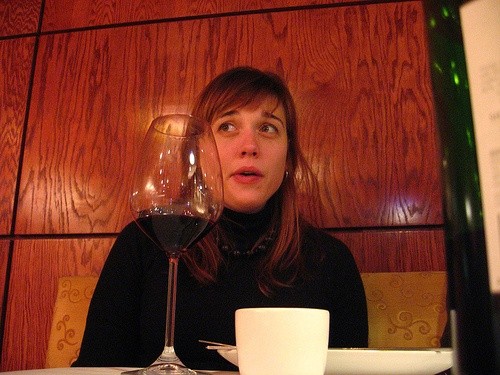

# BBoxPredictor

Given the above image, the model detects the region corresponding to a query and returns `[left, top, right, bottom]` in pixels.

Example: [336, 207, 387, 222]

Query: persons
[71, 66, 368, 372]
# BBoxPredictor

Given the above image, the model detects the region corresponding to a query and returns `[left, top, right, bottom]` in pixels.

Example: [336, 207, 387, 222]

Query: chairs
[47, 273, 448, 369]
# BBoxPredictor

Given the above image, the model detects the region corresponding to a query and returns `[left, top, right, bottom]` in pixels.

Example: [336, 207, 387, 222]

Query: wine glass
[120, 113, 224, 374]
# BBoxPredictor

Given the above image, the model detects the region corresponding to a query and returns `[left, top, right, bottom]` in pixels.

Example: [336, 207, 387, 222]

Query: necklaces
[216, 217, 280, 257]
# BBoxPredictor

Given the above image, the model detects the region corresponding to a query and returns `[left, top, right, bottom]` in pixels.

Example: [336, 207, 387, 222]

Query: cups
[235, 308, 330, 375]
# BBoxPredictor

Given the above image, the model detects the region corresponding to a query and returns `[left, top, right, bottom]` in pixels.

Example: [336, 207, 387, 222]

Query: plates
[218, 348, 454, 375]
